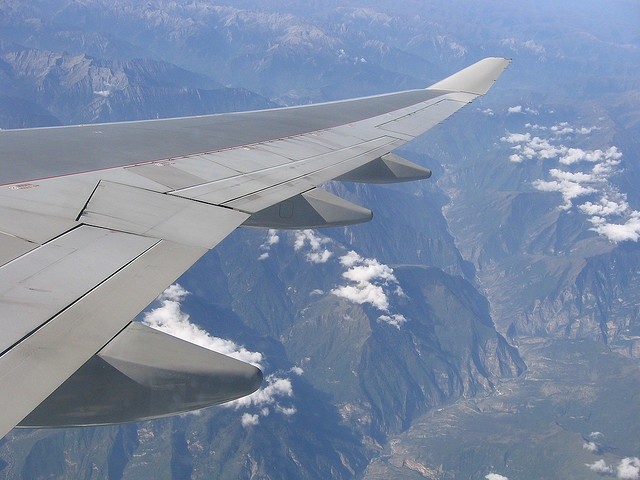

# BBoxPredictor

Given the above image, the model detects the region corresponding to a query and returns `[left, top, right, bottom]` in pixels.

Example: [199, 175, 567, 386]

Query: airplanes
[1, 56, 510, 444]
